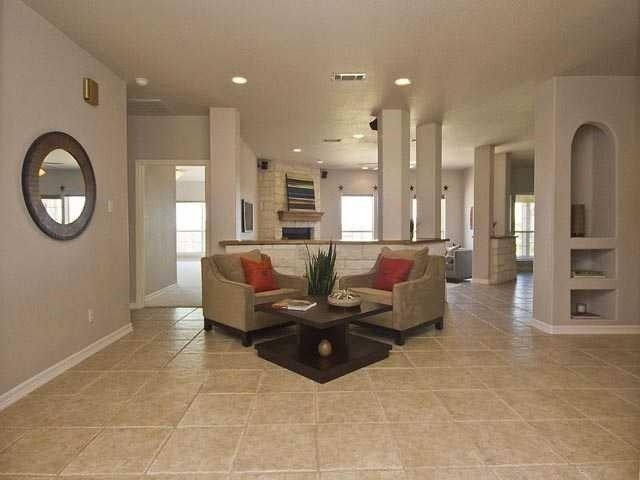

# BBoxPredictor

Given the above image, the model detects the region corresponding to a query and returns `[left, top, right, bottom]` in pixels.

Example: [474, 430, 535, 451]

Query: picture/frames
[243, 200, 253, 233]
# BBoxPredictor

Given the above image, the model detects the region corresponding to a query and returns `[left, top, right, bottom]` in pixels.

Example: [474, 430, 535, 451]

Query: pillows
[371, 256, 414, 291]
[240, 256, 281, 293]
[375, 247, 429, 281]
[214, 249, 262, 284]
[447, 244, 461, 262]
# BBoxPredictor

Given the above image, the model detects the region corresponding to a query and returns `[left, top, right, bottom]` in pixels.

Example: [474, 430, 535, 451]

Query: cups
[576, 303, 586, 313]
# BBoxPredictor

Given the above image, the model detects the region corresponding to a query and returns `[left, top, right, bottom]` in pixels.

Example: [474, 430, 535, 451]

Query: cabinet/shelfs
[490, 236, 517, 285]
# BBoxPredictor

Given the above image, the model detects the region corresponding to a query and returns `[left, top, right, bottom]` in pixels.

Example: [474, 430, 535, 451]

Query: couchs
[444, 247, 473, 282]
[337, 255, 446, 345]
[201, 258, 310, 347]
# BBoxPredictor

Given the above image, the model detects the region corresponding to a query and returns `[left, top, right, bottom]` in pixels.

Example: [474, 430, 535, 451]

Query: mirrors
[21, 131, 97, 240]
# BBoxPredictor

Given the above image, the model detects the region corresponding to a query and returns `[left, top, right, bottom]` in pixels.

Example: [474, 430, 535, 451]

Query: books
[272, 298, 317, 312]
[572, 270, 608, 279]
[572, 313, 601, 319]
